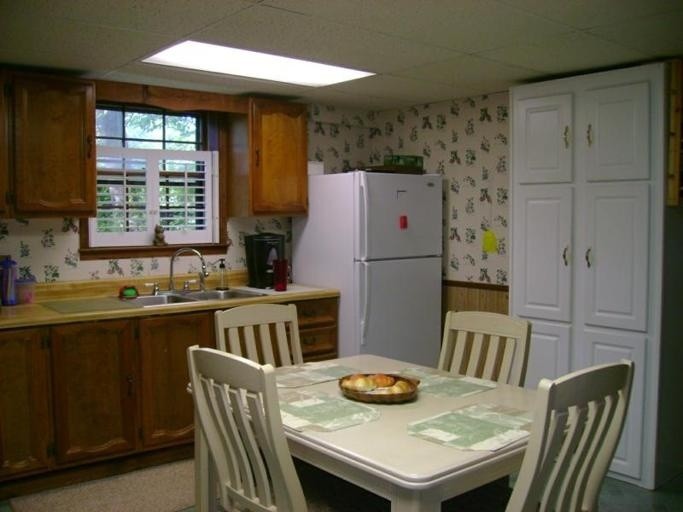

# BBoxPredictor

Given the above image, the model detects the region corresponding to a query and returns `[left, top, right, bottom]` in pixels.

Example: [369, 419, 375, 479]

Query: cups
[0, 256, 18, 306]
[274, 259, 288, 291]
[15, 280, 33, 304]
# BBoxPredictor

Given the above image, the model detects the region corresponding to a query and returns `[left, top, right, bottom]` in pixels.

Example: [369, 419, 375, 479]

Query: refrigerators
[292, 171, 442, 369]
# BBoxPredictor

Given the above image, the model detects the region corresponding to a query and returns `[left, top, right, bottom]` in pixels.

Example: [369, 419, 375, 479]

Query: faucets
[169, 247, 209, 292]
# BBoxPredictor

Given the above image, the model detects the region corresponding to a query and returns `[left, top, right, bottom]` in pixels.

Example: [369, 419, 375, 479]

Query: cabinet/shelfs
[0, 62, 13, 217]
[52, 311, 212, 465]
[506, 59, 669, 491]
[228, 96, 309, 220]
[212, 297, 338, 367]
[11, 68, 97, 217]
[0, 325, 52, 483]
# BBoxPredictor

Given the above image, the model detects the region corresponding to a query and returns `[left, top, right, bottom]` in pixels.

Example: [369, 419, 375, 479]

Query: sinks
[194, 287, 266, 301]
[136, 292, 194, 306]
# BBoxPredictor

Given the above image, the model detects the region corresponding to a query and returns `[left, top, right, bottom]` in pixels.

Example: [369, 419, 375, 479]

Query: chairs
[186, 344, 308, 511]
[214, 302, 304, 368]
[442, 359, 636, 512]
[438, 310, 531, 388]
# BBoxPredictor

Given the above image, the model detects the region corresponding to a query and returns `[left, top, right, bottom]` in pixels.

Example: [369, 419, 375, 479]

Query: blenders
[245, 233, 285, 288]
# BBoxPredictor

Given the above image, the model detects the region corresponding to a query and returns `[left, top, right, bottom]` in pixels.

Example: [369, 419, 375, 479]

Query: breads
[392, 380, 413, 394]
[340, 375, 367, 392]
[367, 373, 395, 387]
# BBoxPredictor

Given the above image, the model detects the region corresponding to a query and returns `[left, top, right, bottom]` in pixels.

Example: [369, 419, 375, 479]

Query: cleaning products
[212, 257, 229, 291]
